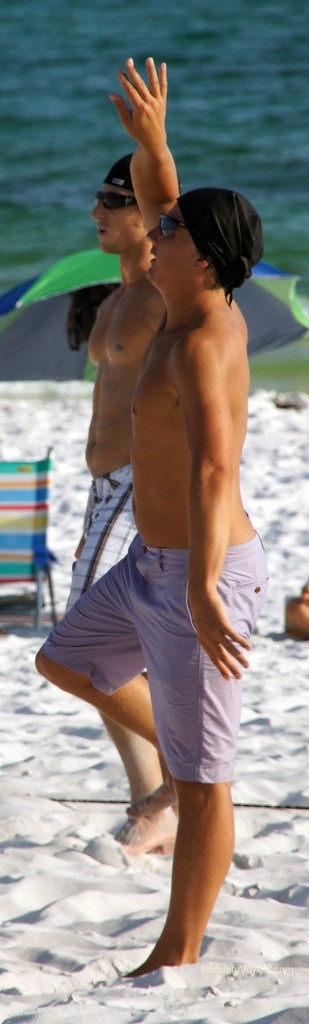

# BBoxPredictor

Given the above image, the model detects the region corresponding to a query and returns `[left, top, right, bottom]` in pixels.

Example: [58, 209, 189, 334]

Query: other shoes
[285, 584, 309, 639]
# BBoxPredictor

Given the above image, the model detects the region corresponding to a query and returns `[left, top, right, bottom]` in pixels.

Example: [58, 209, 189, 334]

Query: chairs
[0, 441, 63, 636]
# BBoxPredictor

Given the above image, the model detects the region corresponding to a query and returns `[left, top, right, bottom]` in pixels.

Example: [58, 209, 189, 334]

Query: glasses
[94, 191, 137, 210]
[159, 213, 185, 238]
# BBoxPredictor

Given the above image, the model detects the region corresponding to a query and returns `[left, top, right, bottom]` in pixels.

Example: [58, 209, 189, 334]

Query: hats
[177, 187, 264, 290]
[103, 152, 181, 197]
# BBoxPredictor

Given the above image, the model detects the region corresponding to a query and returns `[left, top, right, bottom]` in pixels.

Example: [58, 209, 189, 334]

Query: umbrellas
[1, 245, 307, 382]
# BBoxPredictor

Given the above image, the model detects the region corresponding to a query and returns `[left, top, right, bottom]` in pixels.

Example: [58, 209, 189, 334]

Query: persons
[64, 152, 176, 854]
[34, 58, 265, 977]
